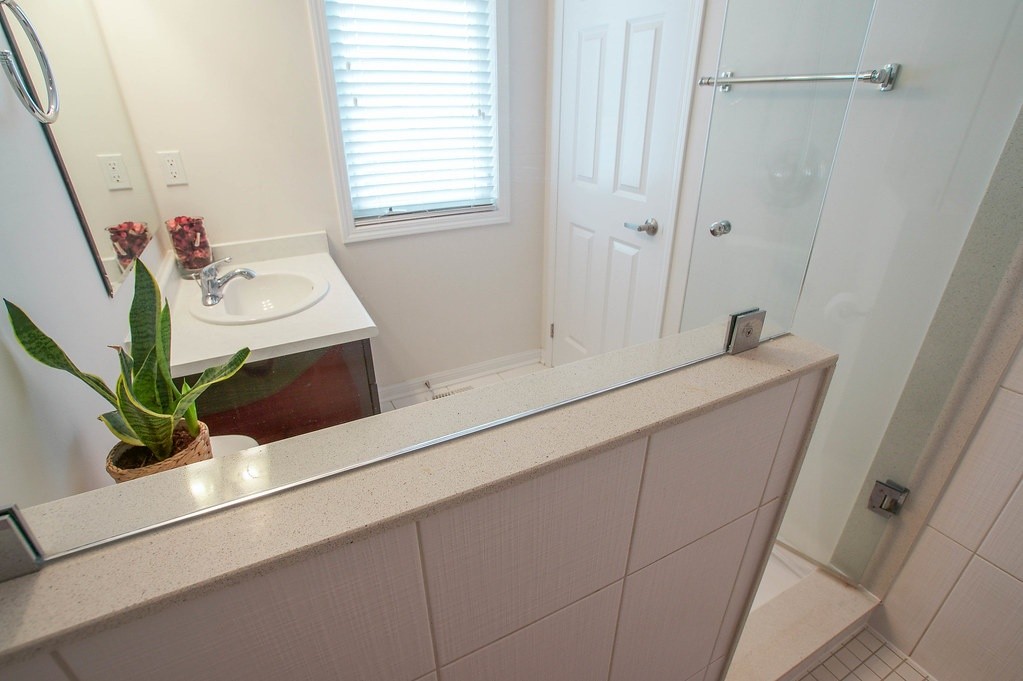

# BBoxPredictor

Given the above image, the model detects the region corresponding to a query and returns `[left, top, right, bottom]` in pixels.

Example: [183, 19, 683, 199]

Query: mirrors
[0, 4, 118, 391]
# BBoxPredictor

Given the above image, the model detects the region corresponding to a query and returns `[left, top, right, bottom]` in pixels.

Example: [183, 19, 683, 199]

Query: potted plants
[4, 256, 253, 486]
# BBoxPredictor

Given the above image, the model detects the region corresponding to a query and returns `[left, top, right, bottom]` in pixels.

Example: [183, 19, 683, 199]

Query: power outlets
[157, 149, 189, 188]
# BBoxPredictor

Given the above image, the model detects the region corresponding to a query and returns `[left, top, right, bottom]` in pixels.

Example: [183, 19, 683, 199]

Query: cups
[163, 215, 215, 281]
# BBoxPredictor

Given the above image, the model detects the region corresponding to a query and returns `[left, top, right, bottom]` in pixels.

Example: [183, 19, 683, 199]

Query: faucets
[197, 256, 258, 306]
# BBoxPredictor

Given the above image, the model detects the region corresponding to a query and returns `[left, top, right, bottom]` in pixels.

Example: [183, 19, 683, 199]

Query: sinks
[187, 267, 331, 326]
